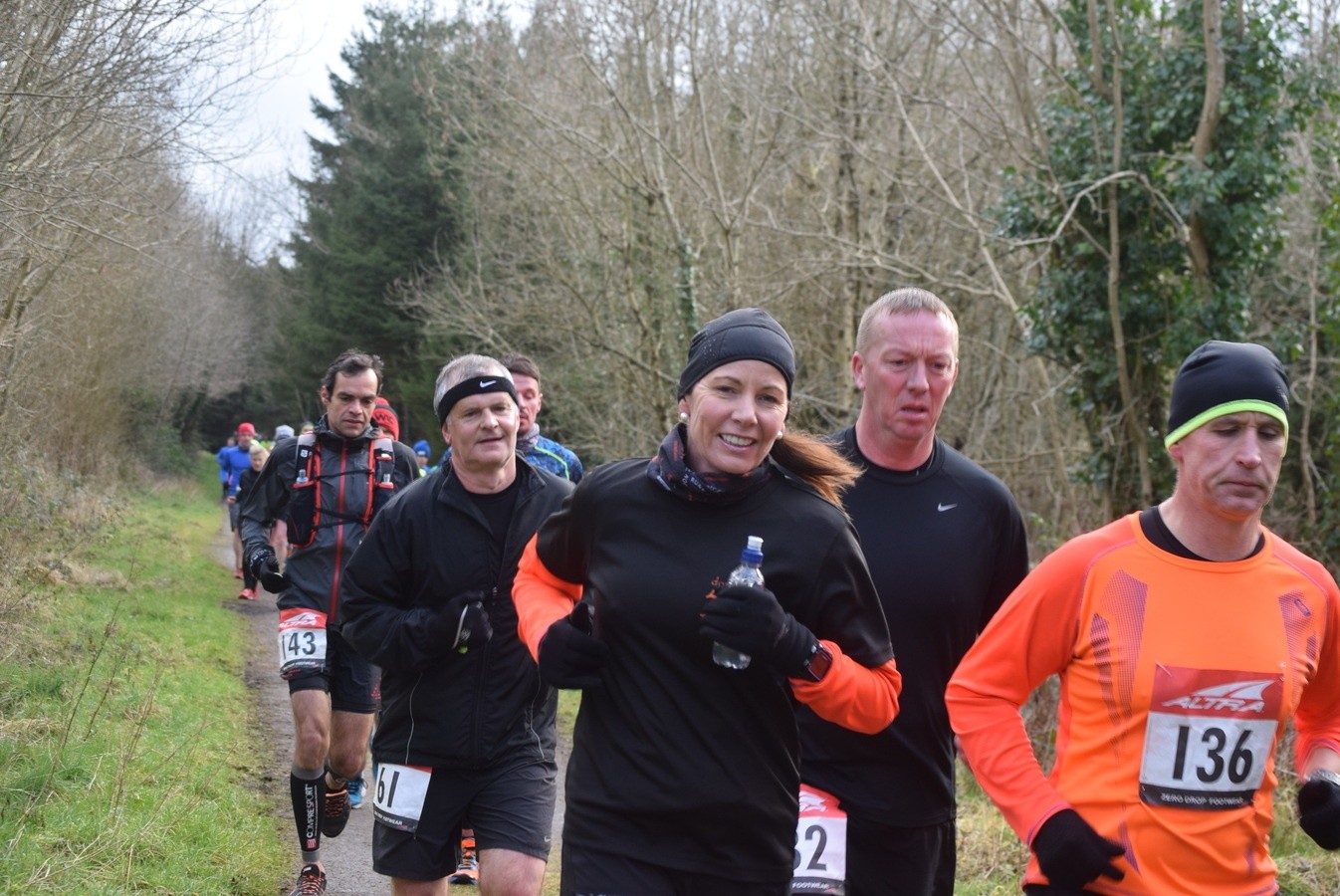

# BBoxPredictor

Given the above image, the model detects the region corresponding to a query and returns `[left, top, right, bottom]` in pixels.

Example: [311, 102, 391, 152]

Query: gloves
[426, 592, 493, 662]
[537, 600, 606, 689]
[1031, 809, 1127, 893]
[697, 585, 815, 672]
[259, 556, 284, 593]
[1296, 779, 1339, 850]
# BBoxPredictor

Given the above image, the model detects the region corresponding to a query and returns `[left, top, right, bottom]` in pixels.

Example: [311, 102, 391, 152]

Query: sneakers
[347, 772, 368, 809]
[286, 864, 327, 896]
[323, 764, 351, 837]
[239, 589, 257, 601]
[234, 567, 244, 579]
[447, 856, 479, 884]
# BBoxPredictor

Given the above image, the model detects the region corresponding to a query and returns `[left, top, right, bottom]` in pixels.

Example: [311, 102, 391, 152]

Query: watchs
[793, 639, 834, 683]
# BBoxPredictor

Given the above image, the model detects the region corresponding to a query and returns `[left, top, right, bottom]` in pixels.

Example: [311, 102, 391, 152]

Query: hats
[238, 422, 255, 437]
[273, 424, 294, 439]
[1161, 339, 1289, 458]
[672, 307, 796, 400]
[370, 397, 399, 442]
[411, 439, 431, 459]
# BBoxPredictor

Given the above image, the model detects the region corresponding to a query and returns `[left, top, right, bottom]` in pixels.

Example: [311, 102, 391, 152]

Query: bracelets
[1304, 767, 1340, 786]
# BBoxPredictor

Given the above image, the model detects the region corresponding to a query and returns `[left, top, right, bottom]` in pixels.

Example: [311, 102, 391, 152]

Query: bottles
[711, 533, 764, 670]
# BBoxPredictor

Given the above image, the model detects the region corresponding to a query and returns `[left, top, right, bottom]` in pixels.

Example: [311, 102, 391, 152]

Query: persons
[260, 435, 272, 450]
[225, 423, 255, 579]
[412, 440, 433, 478]
[944, 342, 1340, 896]
[435, 349, 583, 484]
[508, 307, 901, 895]
[370, 396, 399, 441]
[819, 287, 1031, 896]
[227, 445, 269, 598]
[238, 350, 422, 895]
[217, 437, 236, 502]
[337, 352, 594, 896]
[297, 421, 314, 434]
[274, 425, 294, 446]
